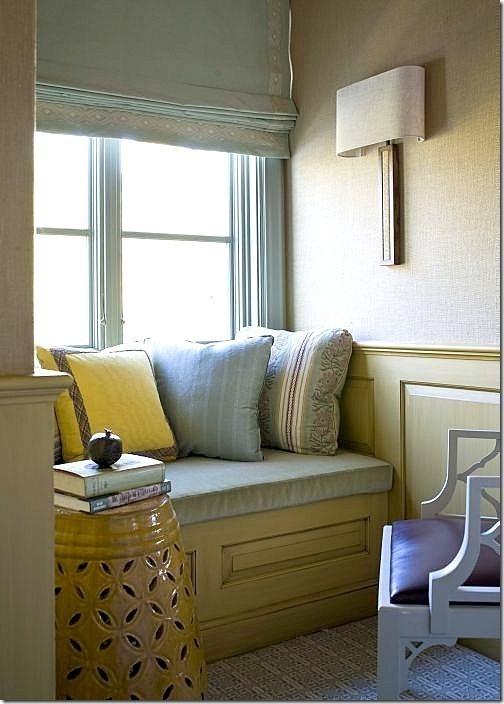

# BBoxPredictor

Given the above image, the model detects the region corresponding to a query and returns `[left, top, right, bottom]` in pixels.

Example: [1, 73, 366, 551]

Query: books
[53, 451, 164, 497]
[52, 479, 171, 511]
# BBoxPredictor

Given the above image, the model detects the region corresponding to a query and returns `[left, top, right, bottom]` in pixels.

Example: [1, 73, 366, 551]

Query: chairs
[374, 429, 502, 703]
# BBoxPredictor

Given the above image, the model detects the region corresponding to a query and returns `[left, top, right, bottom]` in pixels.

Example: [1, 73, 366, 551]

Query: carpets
[201, 614, 502, 703]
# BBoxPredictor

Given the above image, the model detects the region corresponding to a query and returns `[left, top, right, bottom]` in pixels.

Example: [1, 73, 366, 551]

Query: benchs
[54, 443, 394, 663]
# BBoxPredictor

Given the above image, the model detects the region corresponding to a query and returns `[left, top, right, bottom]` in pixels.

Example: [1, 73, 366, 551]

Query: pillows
[35, 338, 177, 463]
[234, 326, 355, 456]
[143, 335, 275, 462]
